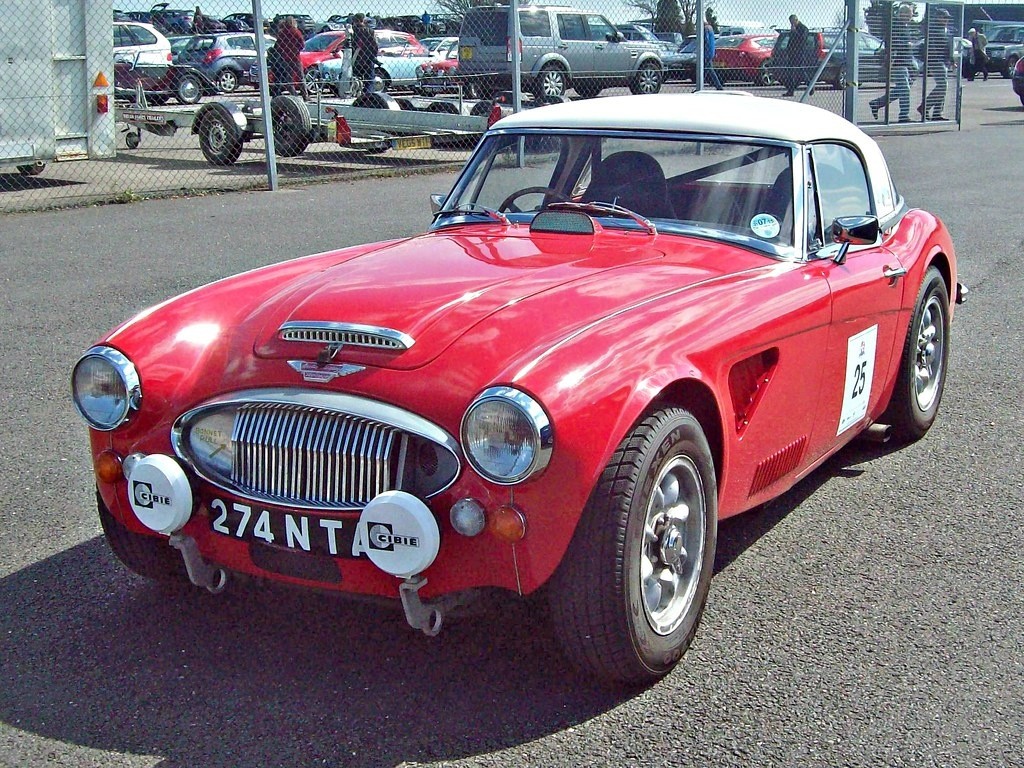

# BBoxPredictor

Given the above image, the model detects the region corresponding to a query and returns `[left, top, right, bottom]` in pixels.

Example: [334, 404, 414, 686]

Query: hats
[968, 28, 975, 33]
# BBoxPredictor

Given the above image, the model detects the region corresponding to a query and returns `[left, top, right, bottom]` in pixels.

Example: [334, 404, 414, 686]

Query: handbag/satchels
[974, 49, 988, 64]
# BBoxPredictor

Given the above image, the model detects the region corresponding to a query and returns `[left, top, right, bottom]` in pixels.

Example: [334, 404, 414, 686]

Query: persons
[967, 28, 989, 81]
[784, 14, 815, 97]
[193, 6, 202, 22]
[917, 9, 958, 120]
[869, 4, 917, 122]
[422, 11, 432, 34]
[703, 21, 723, 90]
[347, 13, 378, 93]
[274, 16, 310, 101]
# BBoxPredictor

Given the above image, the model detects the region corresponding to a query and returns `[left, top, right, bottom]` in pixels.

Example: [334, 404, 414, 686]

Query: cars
[180, 32, 273, 94]
[408, 37, 464, 96]
[113, 2, 464, 39]
[616, 23, 718, 82]
[692, 32, 783, 84]
[250, 28, 421, 95]
[320, 46, 442, 97]
[418, 37, 460, 60]
[718, 25, 777, 35]
[111, 21, 172, 66]
[967, 24, 1024, 80]
[911, 36, 978, 73]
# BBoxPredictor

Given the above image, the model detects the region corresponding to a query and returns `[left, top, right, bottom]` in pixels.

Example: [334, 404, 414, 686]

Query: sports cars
[67, 90, 968, 685]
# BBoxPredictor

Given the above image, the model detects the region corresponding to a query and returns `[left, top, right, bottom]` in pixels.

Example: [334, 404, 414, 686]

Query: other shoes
[968, 77, 974, 81]
[897, 118, 916, 122]
[917, 106, 930, 120]
[809, 86, 814, 95]
[783, 91, 793, 96]
[303, 96, 310, 101]
[869, 100, 879, 120]
[930, 115, 949, 121]
[983, 75, 988, 81]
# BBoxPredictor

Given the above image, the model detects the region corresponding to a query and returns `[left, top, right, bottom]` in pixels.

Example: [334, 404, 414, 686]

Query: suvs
[769, 26, 921, 89]
[454, 5, 666, 100]
